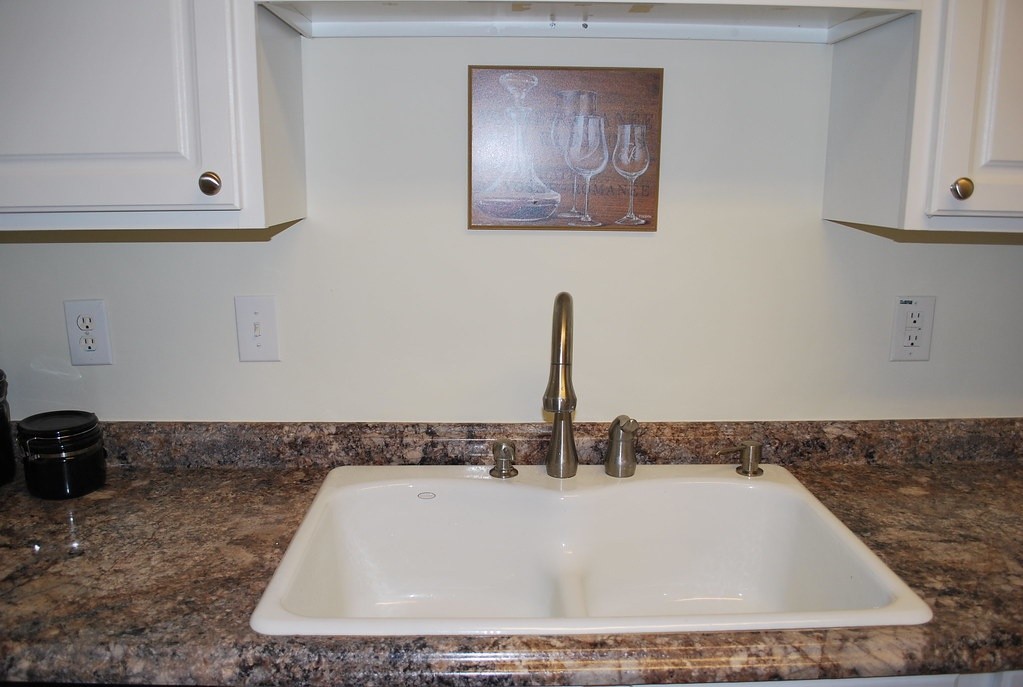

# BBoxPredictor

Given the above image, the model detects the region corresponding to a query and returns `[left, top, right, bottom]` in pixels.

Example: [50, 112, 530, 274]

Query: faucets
[542, 291, 580, 478]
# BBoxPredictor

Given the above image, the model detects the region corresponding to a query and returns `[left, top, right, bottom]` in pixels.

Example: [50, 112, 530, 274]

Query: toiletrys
[0, 368, 15, 486]
[17, 410, 105, 500]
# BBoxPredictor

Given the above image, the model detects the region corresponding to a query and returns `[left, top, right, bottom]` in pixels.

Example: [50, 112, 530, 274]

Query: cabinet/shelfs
[822, 0, 1023, 232]
[0, 0, 311, 245]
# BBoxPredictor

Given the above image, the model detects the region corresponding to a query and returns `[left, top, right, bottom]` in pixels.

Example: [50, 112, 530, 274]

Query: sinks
[249, 464, 934, 639]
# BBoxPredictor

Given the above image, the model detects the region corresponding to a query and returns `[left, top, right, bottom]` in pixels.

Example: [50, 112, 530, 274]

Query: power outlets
[890, 296, 936, 362]
[63, 299, 112, 365]
